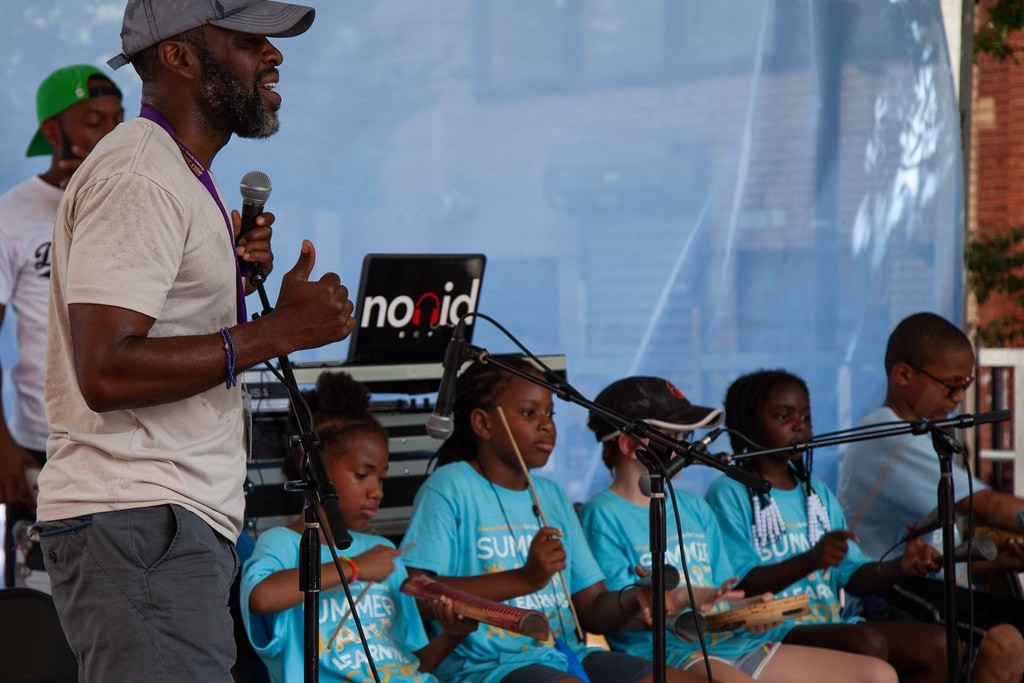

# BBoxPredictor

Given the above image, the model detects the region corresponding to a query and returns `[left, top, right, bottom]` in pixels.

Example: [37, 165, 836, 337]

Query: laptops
[290, 253, 486, 370]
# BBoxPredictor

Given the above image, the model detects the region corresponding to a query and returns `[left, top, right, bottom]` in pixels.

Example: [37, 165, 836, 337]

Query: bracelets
[618, 584, 637, 616]
[220, 326, 237, 390]
[339, 554, 359, 584]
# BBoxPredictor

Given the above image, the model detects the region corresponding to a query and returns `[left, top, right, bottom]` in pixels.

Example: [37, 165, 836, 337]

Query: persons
[397, 354, 674, 683]
[0, 62, 124, 595]
[577, 375, 898, 683]
[703, 369, 964, 682]
[35, 0, 357, 683]
[838, 311, 1024, 683]
[239, 370, 479, 683]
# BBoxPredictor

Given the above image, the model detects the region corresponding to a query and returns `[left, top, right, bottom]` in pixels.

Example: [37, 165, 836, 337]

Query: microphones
[235, 170, 272, 276]
[426, 318, 466, 439]
[638, 428, 723, 497]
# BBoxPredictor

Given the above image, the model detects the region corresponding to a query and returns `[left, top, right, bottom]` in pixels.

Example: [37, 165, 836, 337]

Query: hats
[106, 0, 316, 71]
[25, 65, 123, 157]
[588, 377, 724, 443]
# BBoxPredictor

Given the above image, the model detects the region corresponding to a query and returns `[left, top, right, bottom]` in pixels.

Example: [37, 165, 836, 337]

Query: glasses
[904, 363, 975, 399]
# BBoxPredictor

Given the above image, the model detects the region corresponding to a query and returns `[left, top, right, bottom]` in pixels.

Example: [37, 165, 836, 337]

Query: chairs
[0, 587, 80, 683]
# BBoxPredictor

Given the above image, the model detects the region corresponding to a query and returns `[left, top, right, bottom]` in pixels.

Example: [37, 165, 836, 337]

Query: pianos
[242, 352, 570, 536]
